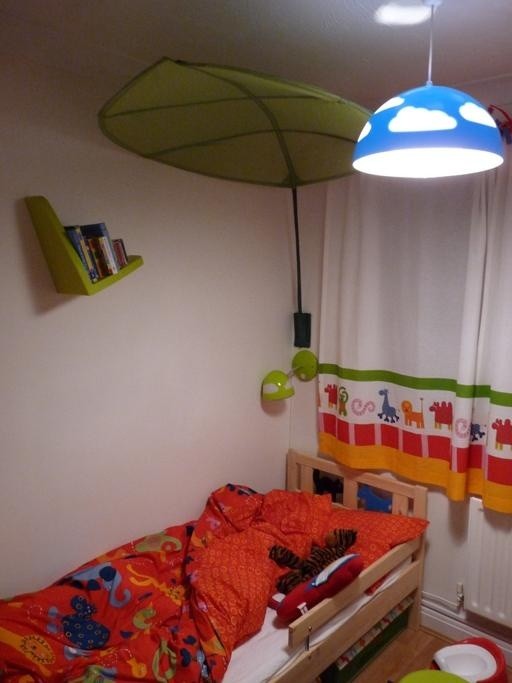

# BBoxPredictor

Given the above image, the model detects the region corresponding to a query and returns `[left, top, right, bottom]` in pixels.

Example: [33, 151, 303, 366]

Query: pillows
[276, 554, 363, 620]
[319, 507, 430, 596]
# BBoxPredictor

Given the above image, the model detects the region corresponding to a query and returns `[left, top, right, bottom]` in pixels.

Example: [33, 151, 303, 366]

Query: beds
[0, 449, 428, 683]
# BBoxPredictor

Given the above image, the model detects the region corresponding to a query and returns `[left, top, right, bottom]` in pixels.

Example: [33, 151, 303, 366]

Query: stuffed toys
[269, 525, 357, 594]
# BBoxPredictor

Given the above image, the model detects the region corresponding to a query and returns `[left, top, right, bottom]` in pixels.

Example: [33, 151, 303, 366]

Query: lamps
[351, 0, 504, 179]
[261, 350, 318, 401]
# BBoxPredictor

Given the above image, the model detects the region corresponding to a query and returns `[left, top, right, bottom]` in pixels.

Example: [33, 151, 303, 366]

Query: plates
[433, 644, 497, 682]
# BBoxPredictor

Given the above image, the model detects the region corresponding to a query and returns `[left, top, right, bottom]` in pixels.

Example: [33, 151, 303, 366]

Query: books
[64, 221, 129, 284]
